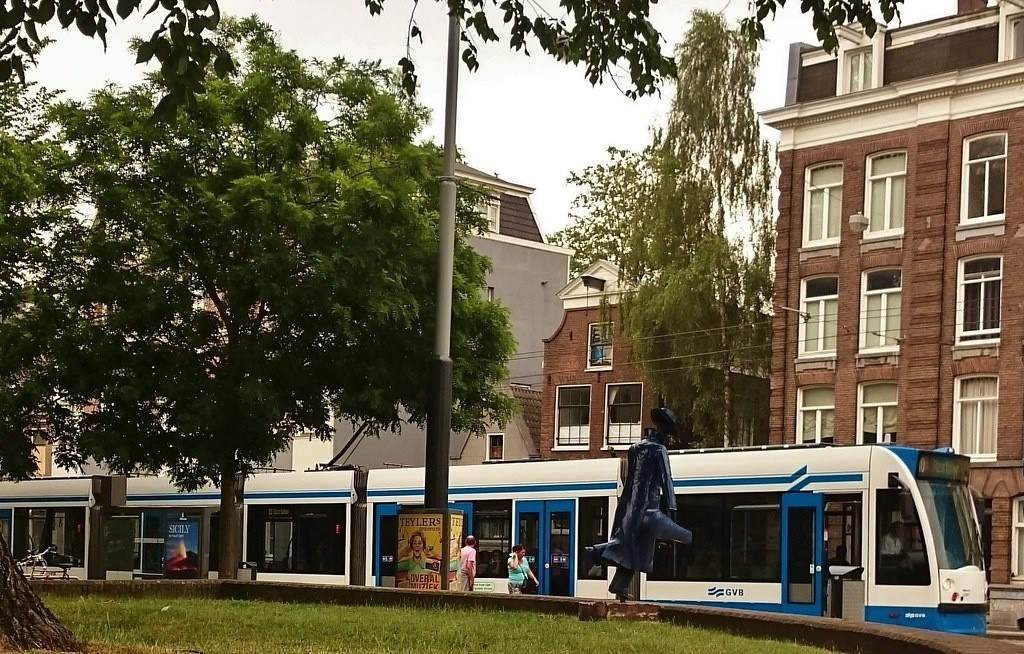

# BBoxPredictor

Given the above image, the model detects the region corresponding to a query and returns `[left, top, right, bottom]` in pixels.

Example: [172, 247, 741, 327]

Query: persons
[507, 543, 540, 595]
[587, 551, 603, 575]
[458, 534, 477, 592]
[601, 406, 679, 599]
[879, 521, 912, 565]
[826, 544, 851, 569]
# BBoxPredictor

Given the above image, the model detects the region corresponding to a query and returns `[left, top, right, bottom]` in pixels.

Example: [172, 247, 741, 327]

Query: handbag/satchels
[518, 564, 529, 594]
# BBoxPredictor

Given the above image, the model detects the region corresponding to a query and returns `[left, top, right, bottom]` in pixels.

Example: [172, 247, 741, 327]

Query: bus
[0, 445, 989, 636]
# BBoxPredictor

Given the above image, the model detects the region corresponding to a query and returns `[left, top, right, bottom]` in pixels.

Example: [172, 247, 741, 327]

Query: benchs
[23, 576, 79, 580]
[23, 574, 51, 580]
[131, 572, 164, 580]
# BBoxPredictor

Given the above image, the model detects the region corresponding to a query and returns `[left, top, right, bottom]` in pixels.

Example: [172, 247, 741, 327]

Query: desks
[33, 567, 64, 580]
